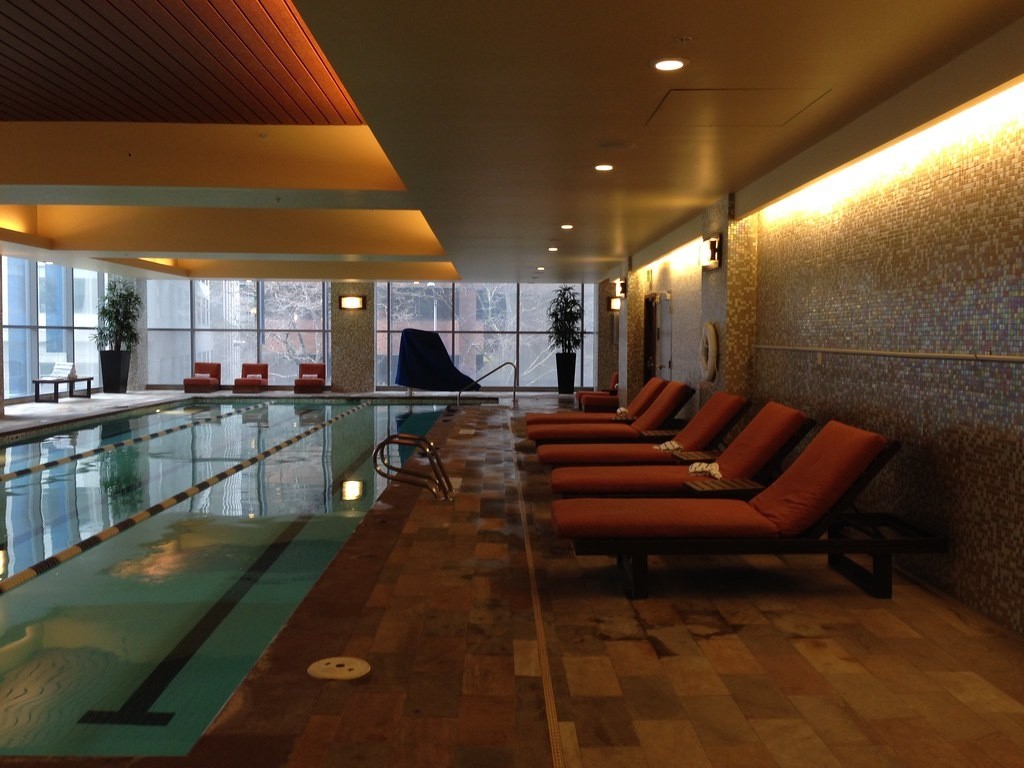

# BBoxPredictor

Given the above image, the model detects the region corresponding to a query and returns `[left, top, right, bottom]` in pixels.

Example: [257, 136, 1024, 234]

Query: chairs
[234, 363, 269, 393]
[294, 363, 326, 394]
[241, 406, 269, 427]
[525, 381, 697, 447]
[184, 363, 221, 394]
[524, 377, 668, 426]
[295, 405, 326, 426]
[183, 404, 223, 425]
[536, 391, 749, 469]
[552, 419, 950, 600]
[576, 373, 618, 409]
[551, 400, 818, 498]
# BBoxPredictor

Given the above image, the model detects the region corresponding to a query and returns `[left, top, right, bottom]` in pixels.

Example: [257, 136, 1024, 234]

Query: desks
[683, 477, 765, 500]
[672, 450, 720, 464]
[641, 429, 680, 443]
[32, 376, 95, 403]
[611, 416, 637, 424]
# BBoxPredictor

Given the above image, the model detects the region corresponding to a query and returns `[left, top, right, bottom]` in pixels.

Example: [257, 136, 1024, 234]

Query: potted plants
[100, 420, 145, 520]
[88, 275, 143, 393]
[548, 283, 585, 394]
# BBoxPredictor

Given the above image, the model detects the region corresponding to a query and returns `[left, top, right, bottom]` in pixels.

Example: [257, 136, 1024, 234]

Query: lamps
[608, 295, 621, 311]
[699, 238, 718, 266]
[615, 280, 626, 297]
[339, 295, 367, 310]
[340, 480, 366, 500]
